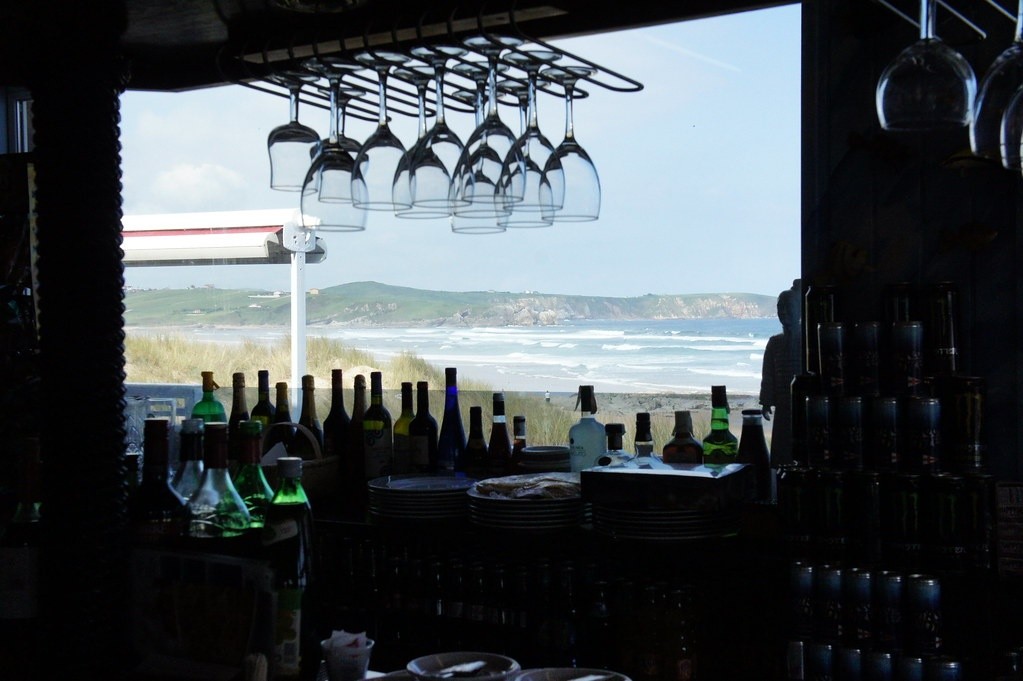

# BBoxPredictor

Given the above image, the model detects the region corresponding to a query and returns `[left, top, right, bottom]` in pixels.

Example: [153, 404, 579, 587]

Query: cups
[320, 637, 376, 681]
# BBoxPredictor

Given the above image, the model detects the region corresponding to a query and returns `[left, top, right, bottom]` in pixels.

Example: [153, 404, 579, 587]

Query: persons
[544, 391, 551, 403]
[759, 279, 802, 471]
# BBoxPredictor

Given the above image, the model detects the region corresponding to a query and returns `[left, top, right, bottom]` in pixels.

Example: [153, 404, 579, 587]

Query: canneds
[777, 280, 997, 681]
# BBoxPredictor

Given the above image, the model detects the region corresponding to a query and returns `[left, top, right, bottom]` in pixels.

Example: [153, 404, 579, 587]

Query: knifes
[431, 661, 488, 679]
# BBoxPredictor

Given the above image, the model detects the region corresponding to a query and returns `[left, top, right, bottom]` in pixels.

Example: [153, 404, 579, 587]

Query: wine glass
[875, 1, 1023, 170]
[269, 33, 602, 232]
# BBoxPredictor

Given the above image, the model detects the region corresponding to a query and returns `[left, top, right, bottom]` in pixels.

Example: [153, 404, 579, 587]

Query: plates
[366, 475, 477, 519]
[467, 472, 596, 530]
[539, 668, 631, 681]
[407, 652, 521, 681]
[591, 506, 743, 540]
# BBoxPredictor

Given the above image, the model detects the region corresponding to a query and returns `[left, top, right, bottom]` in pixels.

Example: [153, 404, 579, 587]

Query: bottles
[664, 414, 704, 464]
[410, 383, 438, 473]
[704, 385, 738, 464]
[569, 386, 663, 474]
[436, 368, 467, 476]
[0, 436, 45, 625]
[323, 370, 349, 512]
[364, 372, 393, 474]
[512, 416, 528, 470]
[393, 383, 414, 476]
[343, 377, 371, 512]
[126, 371, 321, 681]
[489, 393, 509, 473]
[737, 409, 772, 546]
[325, 533, 700, 681]
[466, 407, 489, 472]
